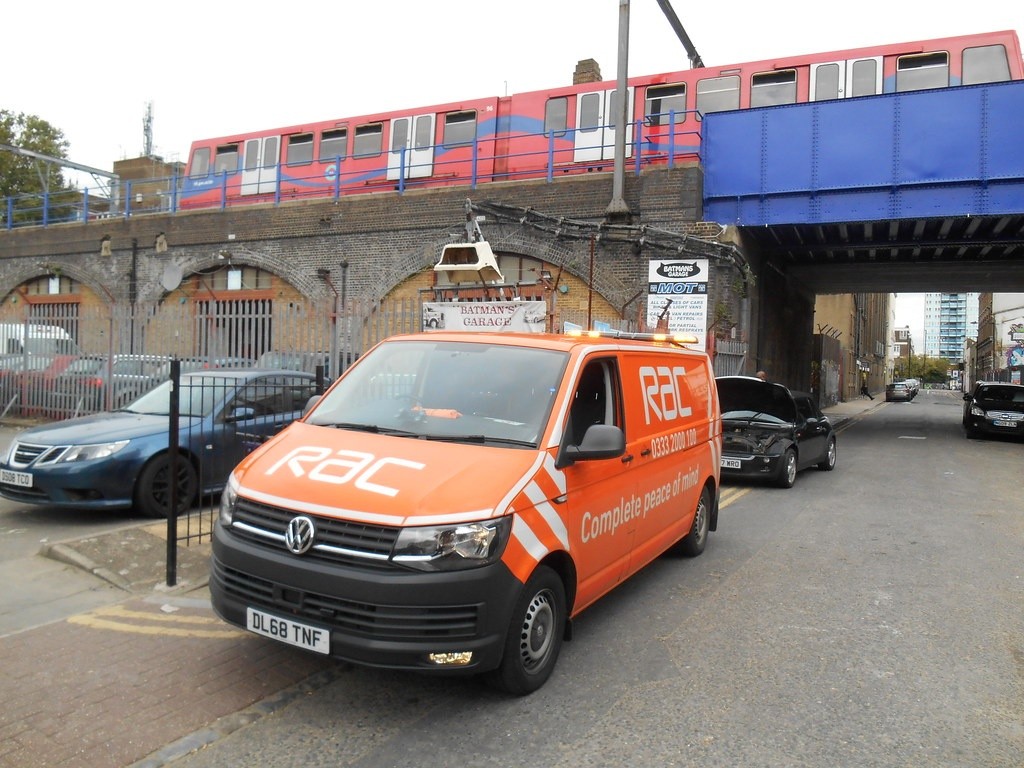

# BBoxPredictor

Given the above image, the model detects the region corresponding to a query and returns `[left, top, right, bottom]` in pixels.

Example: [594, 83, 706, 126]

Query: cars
[250, 349, 362, 382]
[51, 353, 176, 410]
[143, 355, 255, 390]
[961, 380, 1024, 443]
[0, 368, 340, 517]
[710, 376, 838, 487]
[1, 353, 79, 419]
[885, 378, 919, 402]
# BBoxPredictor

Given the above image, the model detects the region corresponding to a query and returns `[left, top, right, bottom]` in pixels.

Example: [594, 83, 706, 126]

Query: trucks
[0, 323, 81, 356]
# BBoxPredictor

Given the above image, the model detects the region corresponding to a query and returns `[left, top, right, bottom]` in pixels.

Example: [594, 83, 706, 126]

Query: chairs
[569, 383, 607, 446]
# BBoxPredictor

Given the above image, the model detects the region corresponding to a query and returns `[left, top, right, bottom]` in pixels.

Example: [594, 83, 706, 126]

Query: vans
[206, 329, 724, 697]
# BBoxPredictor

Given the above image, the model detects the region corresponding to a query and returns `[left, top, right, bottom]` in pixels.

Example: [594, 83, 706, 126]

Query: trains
[176, 28, 1024, 211]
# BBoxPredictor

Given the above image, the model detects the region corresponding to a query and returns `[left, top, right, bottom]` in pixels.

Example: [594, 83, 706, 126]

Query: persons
[862, 383, 875, 400]
[756, 371, 766, 381]
